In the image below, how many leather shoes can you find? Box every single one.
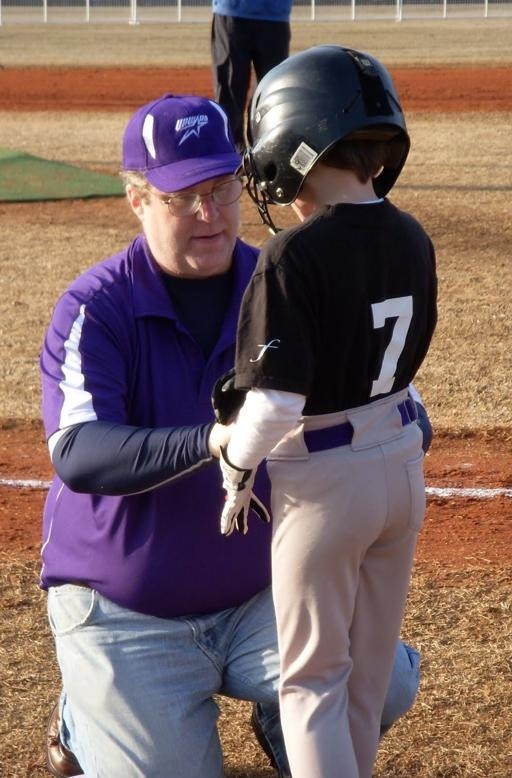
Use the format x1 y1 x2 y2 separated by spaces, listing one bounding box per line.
43 696 84 778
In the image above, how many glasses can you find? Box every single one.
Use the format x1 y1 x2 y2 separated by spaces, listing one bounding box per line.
141 177 249 220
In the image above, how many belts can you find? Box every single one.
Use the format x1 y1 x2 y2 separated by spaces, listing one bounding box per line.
304 396 418 451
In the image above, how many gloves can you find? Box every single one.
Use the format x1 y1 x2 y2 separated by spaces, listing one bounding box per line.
213 443 273 540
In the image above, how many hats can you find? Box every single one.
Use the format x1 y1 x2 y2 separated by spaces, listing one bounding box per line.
118 91 245 195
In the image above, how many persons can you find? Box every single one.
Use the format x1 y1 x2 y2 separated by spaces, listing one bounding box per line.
210 0 292 175
38 96 432 778
210 47 438 778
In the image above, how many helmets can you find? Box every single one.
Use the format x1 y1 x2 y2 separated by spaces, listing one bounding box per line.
240 44 414 208
